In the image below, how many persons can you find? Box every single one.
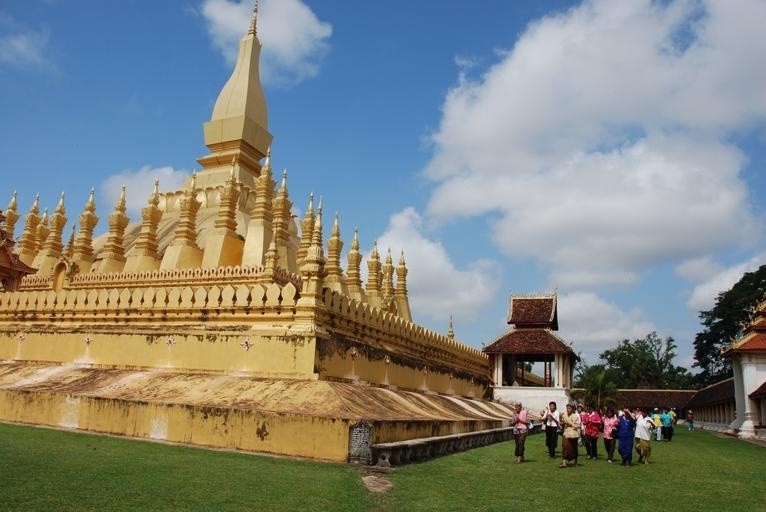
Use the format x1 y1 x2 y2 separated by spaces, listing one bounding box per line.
540 402 677 468
510 402 530 464
686 409 695 431
